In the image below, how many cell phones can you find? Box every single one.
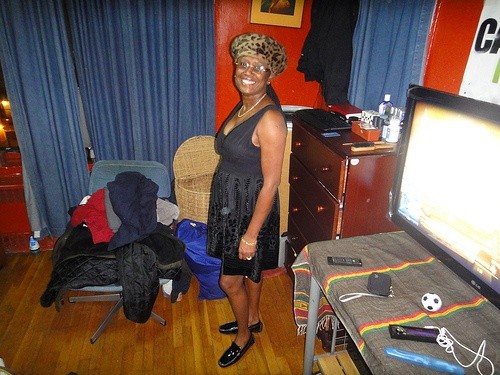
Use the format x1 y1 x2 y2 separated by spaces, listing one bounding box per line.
388 325 446 344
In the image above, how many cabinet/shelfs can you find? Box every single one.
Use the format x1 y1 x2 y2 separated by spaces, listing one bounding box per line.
0 152 94 254
291 230 500 375
284 116 398 284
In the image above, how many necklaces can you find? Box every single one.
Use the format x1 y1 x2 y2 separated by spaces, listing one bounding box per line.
238 93 267 117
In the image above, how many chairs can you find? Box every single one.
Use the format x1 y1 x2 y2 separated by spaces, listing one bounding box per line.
67 159 183 344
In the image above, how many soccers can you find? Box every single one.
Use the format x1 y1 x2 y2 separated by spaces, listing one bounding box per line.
421 292 442 311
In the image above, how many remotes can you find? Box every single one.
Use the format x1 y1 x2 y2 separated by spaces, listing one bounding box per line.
327 256 362 267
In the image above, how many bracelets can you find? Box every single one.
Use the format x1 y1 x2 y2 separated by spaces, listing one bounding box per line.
242 236 257 245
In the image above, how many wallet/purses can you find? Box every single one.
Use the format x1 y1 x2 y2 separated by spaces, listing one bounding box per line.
366 270 391 295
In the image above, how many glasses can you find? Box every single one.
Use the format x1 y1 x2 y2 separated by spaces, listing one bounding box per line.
235 59 271 74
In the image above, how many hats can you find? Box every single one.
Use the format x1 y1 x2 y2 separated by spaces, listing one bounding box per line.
230 32 288 79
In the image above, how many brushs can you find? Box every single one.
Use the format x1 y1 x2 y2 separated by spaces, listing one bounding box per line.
351 142 394 151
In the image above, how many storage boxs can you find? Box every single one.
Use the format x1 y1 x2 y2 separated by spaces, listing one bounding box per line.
352 121 379 141
361 110 380 125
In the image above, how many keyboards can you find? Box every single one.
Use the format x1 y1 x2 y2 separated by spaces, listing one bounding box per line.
294 108 353 131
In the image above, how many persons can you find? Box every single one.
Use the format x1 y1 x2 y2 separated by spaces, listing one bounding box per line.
204 32 290 368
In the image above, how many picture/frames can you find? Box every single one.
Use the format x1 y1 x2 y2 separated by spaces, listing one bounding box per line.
248 0 304 29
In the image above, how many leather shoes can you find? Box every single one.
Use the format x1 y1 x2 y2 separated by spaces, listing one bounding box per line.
218 331 256 367
218 319 263 334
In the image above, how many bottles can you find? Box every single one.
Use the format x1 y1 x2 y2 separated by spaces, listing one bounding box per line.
28 235 40 254
372 94 401 143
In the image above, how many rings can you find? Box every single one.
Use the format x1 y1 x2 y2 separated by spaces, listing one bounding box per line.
247 257 252 260
243 257 246 258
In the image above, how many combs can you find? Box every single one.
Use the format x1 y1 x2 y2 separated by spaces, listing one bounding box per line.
350 142 393 152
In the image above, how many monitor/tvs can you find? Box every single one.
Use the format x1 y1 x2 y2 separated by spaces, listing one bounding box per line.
386 83 500 311
